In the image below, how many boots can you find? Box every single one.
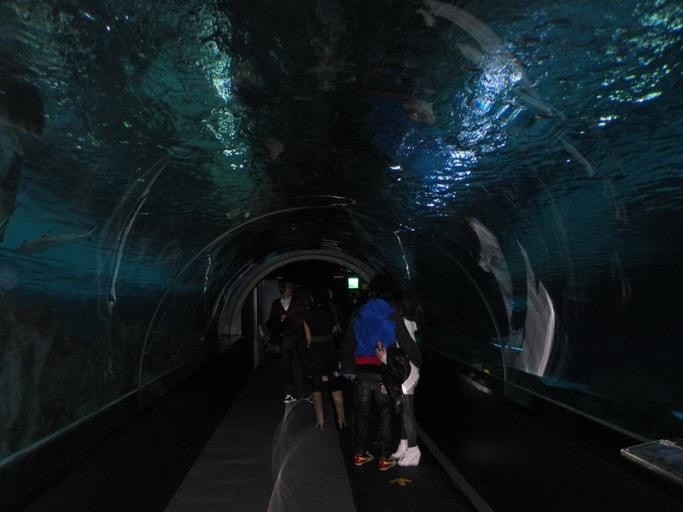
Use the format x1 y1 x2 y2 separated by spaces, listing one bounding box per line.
392 439 421 466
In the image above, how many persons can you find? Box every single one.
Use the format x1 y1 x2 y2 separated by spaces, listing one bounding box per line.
266 267 424 470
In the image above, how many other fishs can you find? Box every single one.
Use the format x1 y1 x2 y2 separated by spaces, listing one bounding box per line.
11 226 100 258
311 0 683 197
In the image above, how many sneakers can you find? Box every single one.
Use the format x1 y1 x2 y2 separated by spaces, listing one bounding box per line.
283 394 296 403
379 457 397 470
355 453 374 465
304 394 314 404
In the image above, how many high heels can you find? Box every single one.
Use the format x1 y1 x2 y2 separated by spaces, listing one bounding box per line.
338 416 347 428
315 417 324 431
